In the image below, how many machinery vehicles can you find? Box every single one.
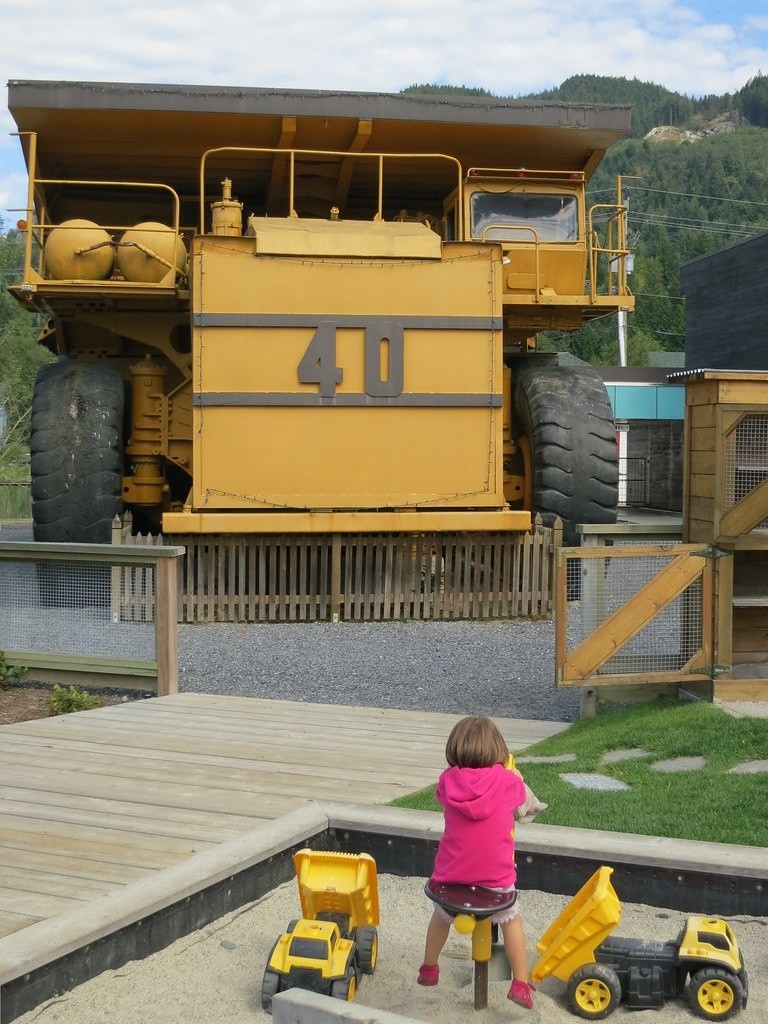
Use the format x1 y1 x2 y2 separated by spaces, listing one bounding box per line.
7 76 639 610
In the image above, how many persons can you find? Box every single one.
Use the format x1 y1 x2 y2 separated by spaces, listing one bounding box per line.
417 717 536 1009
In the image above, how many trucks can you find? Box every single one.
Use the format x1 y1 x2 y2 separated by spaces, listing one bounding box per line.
261 847 382 1005
529 866 750 1021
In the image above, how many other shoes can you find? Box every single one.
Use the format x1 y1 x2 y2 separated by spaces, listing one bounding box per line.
507 979 536 1009
417 964 440 986
